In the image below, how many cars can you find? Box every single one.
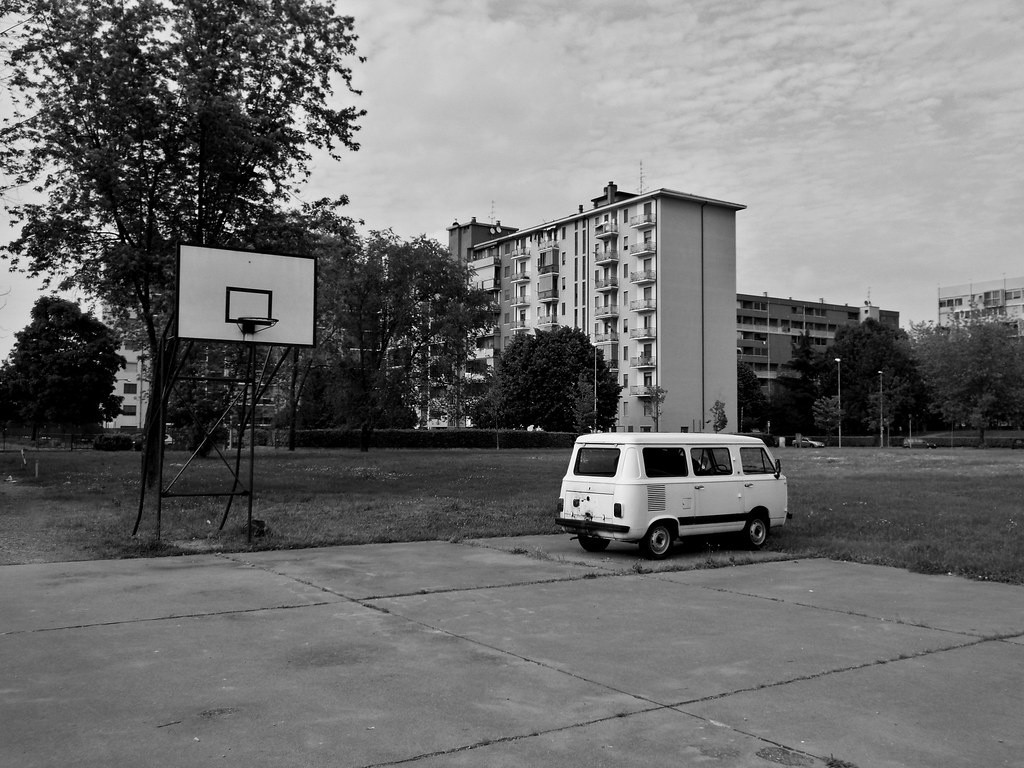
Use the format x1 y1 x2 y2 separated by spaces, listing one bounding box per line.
903 439 937 450
791 436 825 448
165 434 176 446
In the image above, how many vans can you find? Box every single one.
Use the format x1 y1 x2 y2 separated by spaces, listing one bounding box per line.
555 431 793 561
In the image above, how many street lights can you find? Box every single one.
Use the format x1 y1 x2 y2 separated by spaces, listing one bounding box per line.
878 371 885 448
835 358 842 448
591 343 598 433
